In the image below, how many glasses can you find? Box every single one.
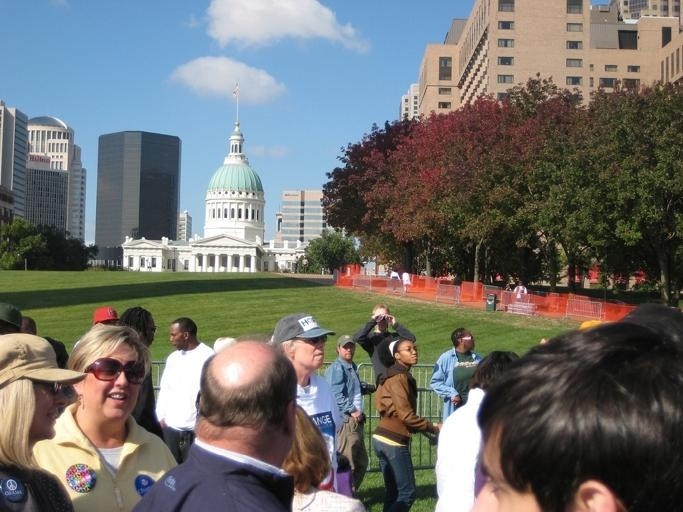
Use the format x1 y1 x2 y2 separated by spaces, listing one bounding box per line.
301 335 326 343
84 358 145 384
34 382 61 395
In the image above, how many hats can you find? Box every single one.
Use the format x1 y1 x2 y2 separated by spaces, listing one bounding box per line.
336 335 355 346
0 301 21 330
92 307 120 324
272 313 336 344
0 333 87 390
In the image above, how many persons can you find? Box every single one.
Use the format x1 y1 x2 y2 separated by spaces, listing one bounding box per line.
477 323 683 512
430 327 483 415
0 299 441 511
504 284 512 295
435 351 521 511
513 281 527 301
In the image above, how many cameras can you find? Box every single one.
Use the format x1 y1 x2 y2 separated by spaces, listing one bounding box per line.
360 381 376 395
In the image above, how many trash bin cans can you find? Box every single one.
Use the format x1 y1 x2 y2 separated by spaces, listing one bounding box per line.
486 294 496 311
